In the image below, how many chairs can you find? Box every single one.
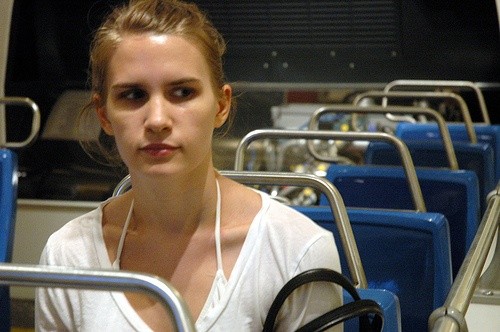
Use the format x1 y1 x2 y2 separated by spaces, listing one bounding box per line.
0 97 44 332
233 80 499 332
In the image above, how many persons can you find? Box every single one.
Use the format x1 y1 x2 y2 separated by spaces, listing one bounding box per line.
34 0 343 332
